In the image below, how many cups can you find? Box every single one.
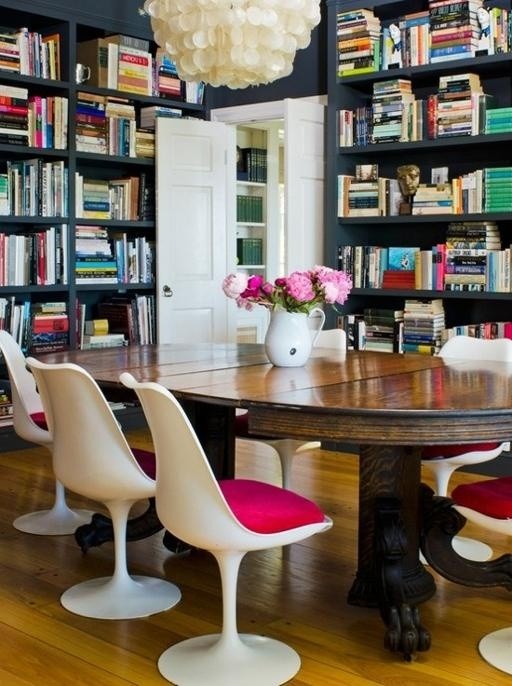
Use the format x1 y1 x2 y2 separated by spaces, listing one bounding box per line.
75 63 92 84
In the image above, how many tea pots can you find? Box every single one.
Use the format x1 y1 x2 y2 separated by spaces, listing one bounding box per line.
265 365 326 411
265 307 326 368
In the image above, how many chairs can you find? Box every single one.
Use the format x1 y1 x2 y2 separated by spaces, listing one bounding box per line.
450 475 512 677
418 335 512 567
0 328 98 536
25 356 182 620
119 372 334 686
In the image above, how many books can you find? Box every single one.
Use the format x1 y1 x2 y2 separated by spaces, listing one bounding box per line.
1 389 14 426
0 159 154 221
1 85 203 159
1 224 152 286
1 293 156 354
338 298 512 356
338 167 512 217
340 73 512 147
336 0 512 76
338 222 512 293
108 401 136 410
0 28 60 80
77 34 203 104
235 146 268 265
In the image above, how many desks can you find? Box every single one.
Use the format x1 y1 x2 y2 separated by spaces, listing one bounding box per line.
0 344 512 663
